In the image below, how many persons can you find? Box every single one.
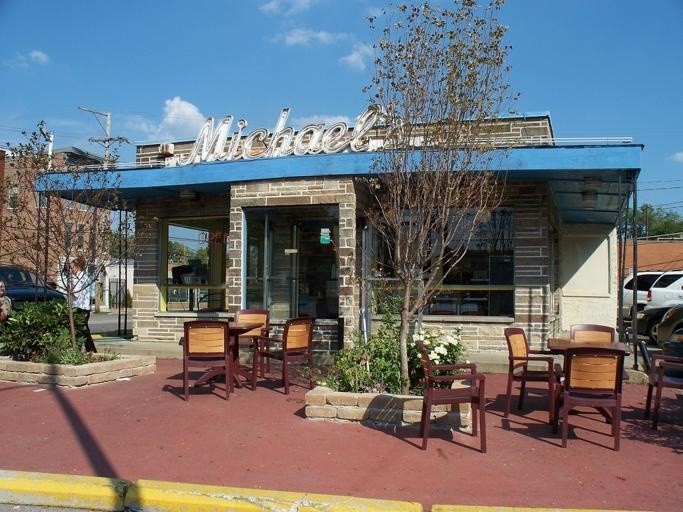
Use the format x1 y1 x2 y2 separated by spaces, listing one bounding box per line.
69 257 96 353
0 281 11 324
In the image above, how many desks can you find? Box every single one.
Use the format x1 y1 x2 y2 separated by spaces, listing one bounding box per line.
548 338 631 424
194 322 264 388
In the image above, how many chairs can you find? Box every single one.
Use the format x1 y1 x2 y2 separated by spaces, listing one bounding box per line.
504 328 558 425
563 324 629 383
429 294 459 315
236 310 273 378
552 347 625 451
179 320 235 401
645 354 683 430
251 318 316 395
415 340 486 453
459 295 489 315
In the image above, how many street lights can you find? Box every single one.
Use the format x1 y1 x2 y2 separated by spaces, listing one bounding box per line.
77 104 111 170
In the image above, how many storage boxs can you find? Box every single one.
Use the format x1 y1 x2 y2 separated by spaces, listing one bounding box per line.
471 278 490 297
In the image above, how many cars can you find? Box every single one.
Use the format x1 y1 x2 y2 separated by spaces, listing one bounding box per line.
0 264 67 309
638 307 671 342
644 270 683 309
623 271 663 321
657 304 683 356
